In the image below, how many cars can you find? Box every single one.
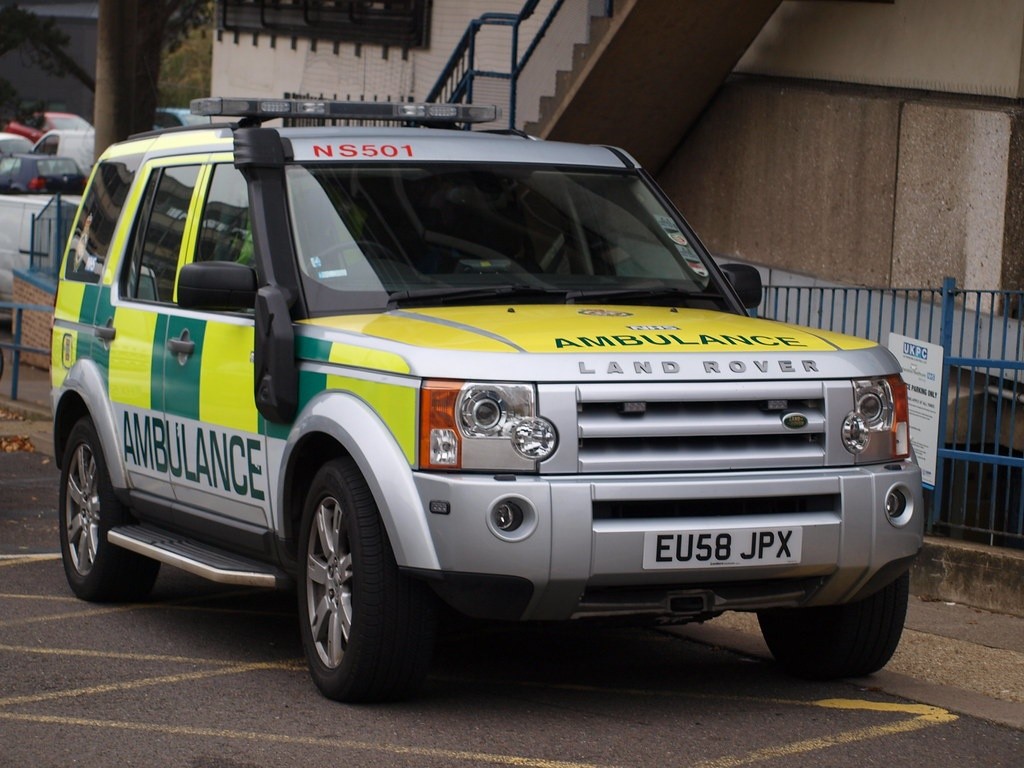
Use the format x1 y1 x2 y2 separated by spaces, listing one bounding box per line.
0 108 212 321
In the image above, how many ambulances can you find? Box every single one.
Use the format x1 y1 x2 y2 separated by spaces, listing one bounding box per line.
49 97 924 703
